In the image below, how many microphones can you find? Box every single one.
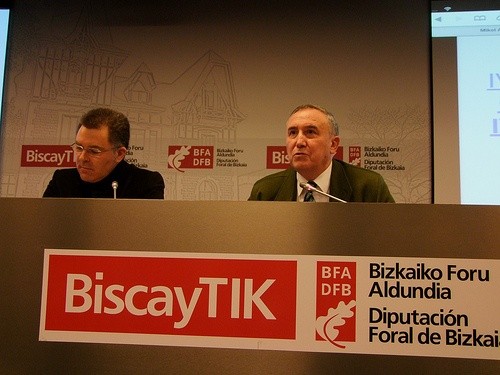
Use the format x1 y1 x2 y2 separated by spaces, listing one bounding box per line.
300 182 347 203
112 181 118 199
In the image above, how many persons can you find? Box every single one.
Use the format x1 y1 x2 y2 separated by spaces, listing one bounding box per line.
41 108 165 200
247 104 395 204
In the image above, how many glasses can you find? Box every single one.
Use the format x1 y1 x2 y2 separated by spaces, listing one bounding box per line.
69 142 121 157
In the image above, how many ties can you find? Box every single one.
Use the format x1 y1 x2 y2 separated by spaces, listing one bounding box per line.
304 181 317 202
